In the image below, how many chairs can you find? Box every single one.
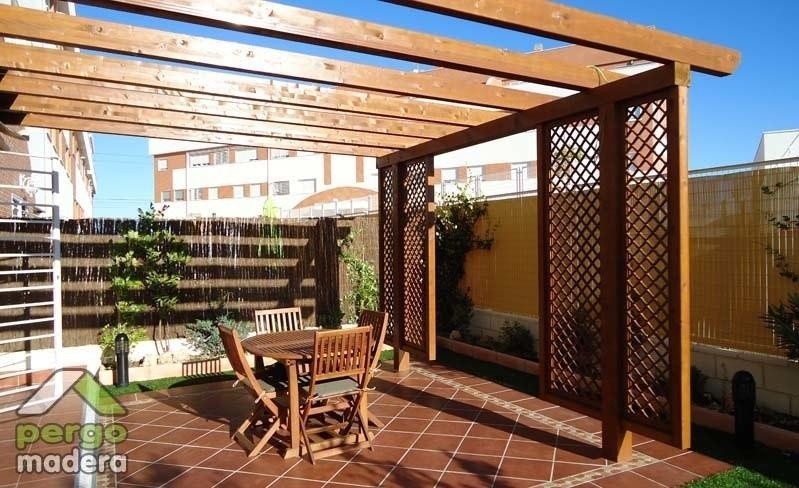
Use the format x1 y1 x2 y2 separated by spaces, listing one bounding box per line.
217 306 390 465
